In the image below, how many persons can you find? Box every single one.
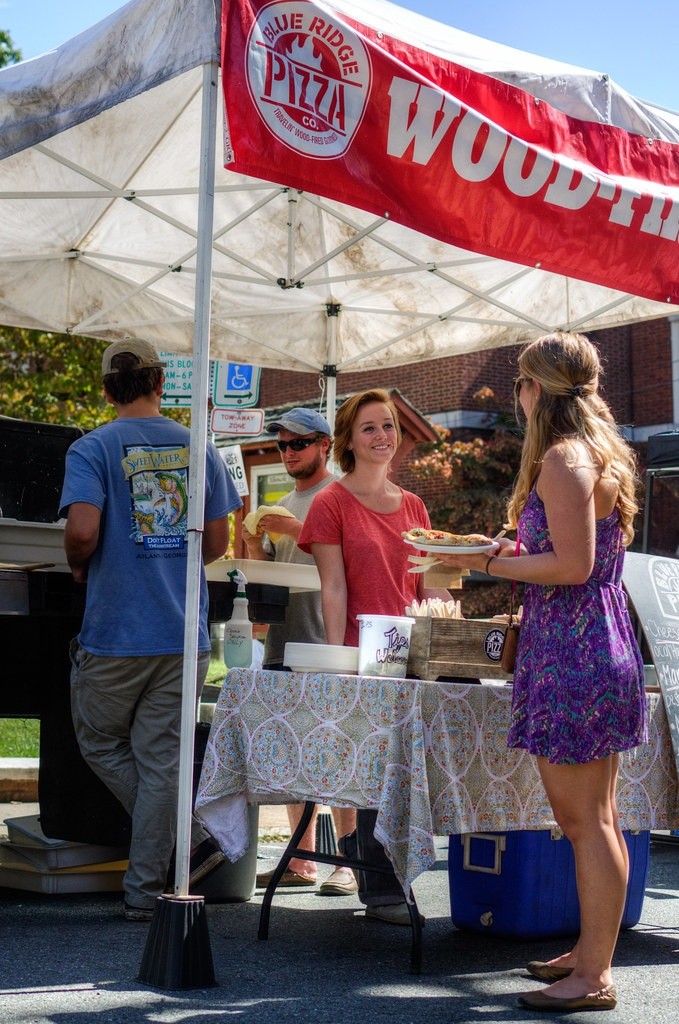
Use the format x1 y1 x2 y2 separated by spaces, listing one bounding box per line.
242 404 359 898
298 392 434 926
436 331 646 1010
59 338 243 920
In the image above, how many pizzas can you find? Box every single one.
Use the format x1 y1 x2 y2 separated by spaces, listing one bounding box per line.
405 527 494 546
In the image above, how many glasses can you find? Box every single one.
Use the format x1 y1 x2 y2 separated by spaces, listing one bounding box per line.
513 377 538 396
278 436 321 453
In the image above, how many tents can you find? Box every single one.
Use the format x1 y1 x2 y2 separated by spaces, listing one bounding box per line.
0 1 679 901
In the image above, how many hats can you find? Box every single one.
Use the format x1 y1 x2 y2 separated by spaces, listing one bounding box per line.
266 407 331 436
100 336 167 375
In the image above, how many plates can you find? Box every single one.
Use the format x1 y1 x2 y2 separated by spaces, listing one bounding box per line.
283 642 358 674
403 538 499 555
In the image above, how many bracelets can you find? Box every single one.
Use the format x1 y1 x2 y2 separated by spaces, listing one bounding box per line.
487 555 496 577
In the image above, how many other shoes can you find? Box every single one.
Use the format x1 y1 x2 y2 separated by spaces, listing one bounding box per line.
528 961 576 981
364 902 424 927
255 864 317 886
124 903 155 921
516 987 617 1014
320 869 357 895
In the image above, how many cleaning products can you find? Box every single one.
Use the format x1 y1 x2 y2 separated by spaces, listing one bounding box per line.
223 571 252 670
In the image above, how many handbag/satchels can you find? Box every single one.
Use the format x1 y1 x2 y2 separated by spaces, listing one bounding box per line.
498 625 521 675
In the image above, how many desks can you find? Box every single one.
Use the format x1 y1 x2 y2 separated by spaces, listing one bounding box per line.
194 665 679 974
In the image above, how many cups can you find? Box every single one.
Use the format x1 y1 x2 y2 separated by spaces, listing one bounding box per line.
355 614 416 679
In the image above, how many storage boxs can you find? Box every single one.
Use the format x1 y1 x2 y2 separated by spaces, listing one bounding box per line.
448 827 650 936
410 616 521 679
